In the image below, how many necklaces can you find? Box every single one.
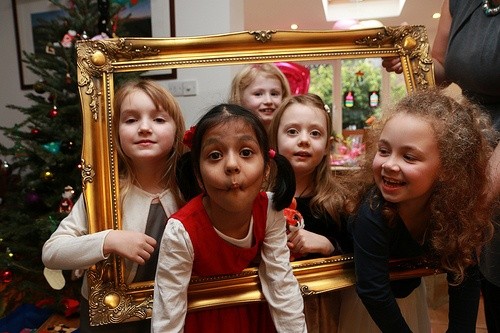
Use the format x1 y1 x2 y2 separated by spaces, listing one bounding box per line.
482 0 500 15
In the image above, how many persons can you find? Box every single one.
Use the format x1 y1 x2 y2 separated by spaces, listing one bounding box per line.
337 92 494 333
41 81 190 333
381 0 500 333
263 94 359 333
230 62 290 129
151 104 309 333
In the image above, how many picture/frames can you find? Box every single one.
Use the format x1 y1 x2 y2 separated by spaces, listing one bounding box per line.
11 0 178 91
72 24 455 326
